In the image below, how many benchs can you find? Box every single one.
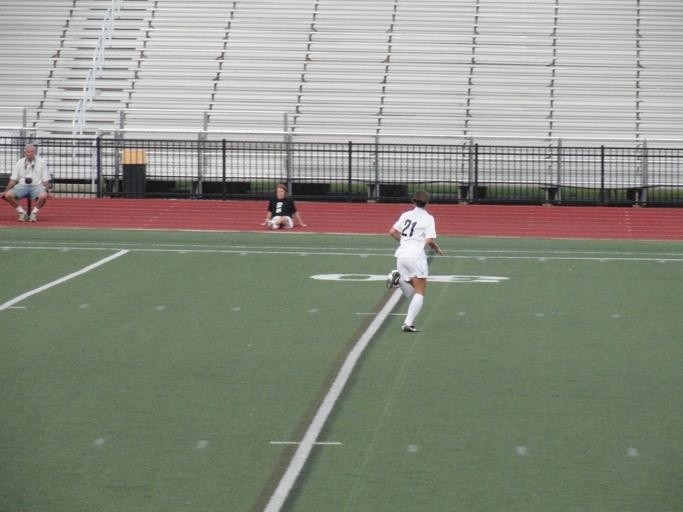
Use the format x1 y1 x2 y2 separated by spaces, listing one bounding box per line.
0 0 683 192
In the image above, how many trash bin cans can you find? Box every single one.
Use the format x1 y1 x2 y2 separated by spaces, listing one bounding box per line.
123 150 147 199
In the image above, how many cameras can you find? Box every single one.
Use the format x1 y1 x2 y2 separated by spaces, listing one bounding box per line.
25 178 32 184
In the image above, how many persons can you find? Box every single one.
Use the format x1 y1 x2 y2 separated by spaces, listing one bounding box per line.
384 190 443 332
0 144 52 223
260 184 308 230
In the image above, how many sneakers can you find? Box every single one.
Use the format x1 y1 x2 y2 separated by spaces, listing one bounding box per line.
387 270 400 288
402 324 416 331
267 220 275 230
18 213 38 222
